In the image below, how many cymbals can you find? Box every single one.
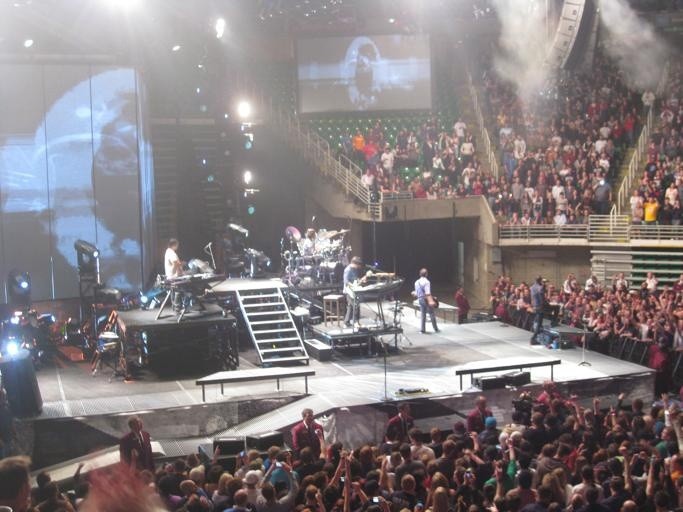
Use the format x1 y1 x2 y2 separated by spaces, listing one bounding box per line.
286 227 301 241
333 229 351 238
318 231 338 241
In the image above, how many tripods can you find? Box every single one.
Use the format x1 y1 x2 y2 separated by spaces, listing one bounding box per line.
386 295 413 348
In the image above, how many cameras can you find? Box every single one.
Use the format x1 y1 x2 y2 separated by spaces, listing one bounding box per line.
372 497 379 502
340 476 344 482
275 462 282 468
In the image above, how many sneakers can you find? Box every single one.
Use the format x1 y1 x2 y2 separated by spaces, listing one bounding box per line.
531 340 540 345
419 331 440 334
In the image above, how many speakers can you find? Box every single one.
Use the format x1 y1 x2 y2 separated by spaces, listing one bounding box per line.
472 371 531 391
0 349 44 418
546 0 603 72
212 430 284 457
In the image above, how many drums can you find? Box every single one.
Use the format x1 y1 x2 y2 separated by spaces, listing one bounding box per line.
319 262 343 280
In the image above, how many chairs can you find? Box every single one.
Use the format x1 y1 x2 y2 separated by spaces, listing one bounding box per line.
309 112 681 378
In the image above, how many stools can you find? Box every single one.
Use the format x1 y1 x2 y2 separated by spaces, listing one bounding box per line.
323 295 346 327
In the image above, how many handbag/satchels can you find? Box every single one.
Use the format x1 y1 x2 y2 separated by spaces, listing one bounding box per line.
424 296 438 309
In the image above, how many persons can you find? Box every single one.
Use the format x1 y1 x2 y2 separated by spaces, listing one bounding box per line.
456 284 470 325
342 256 364 329
164 239 184 317
489 263 681 377
0 380 681 512
415 268 442 334
334 0 683 228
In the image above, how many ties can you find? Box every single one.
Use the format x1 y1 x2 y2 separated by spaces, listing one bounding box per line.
483 414 485 424
404 418 406 435
307 427 312 444
138 434 143 449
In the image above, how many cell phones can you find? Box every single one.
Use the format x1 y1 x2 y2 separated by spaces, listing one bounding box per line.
467 471 470 479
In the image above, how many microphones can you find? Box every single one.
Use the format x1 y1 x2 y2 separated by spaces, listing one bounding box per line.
205 241 213 249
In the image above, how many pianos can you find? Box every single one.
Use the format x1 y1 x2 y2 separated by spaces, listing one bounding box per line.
352 273 406 297
161 273 227 287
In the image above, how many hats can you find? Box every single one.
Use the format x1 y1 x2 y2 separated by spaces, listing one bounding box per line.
189 465 205 481
485 417 496 428
452 421 466 434
242 469 288 484
